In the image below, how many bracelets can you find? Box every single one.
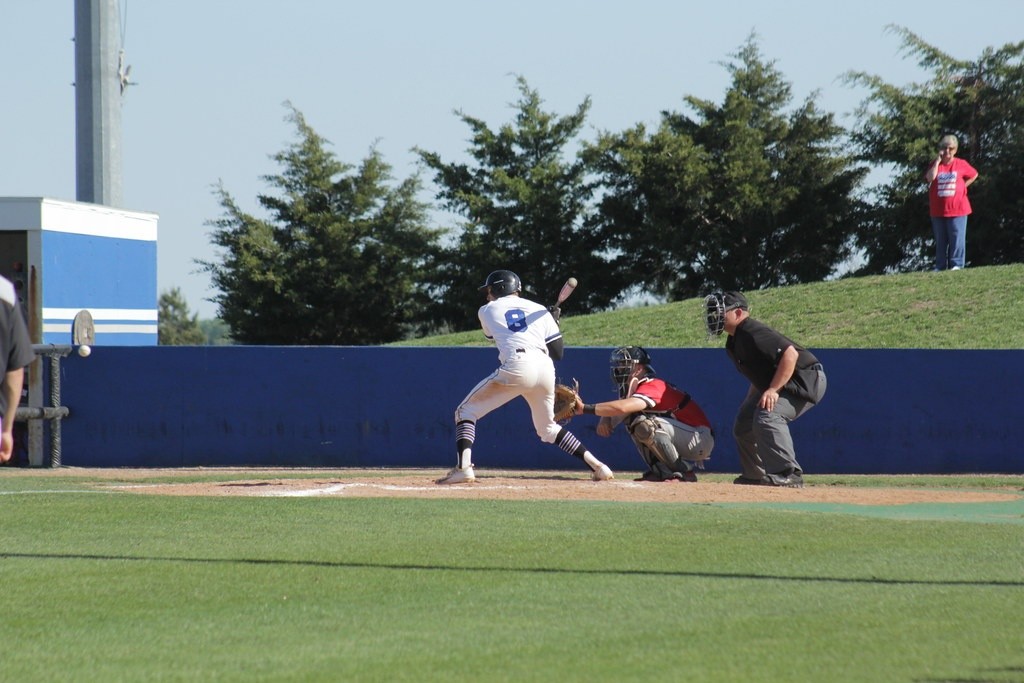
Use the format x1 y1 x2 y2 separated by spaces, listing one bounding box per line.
583 403 596 416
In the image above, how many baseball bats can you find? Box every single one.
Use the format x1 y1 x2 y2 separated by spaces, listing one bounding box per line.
554 277 578 307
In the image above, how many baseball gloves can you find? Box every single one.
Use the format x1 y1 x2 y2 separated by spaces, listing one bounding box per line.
553 376 580 422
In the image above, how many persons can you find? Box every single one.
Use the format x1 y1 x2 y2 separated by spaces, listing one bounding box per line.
701 290 827 489
924 134 978 273
553 346 715 483
0 274 36 461
434 270 614 485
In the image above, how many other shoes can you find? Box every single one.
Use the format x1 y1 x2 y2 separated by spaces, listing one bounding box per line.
931 268 940 272
951 266 960 271
666 456 697 482
634 456 672 481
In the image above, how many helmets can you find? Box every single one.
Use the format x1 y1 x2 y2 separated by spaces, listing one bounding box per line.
478 270 521 298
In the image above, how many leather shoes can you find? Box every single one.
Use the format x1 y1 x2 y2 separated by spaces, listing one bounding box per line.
516 348 547 354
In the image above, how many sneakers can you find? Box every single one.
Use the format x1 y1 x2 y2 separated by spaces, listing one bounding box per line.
760 467 803 488
734 474 770 485
592 464 614 482
435 464 475 484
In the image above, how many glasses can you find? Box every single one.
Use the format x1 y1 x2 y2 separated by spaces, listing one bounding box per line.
942 146 956 150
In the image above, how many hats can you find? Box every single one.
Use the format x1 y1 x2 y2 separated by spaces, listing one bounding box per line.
707 291 748 314
614 346 656 375
